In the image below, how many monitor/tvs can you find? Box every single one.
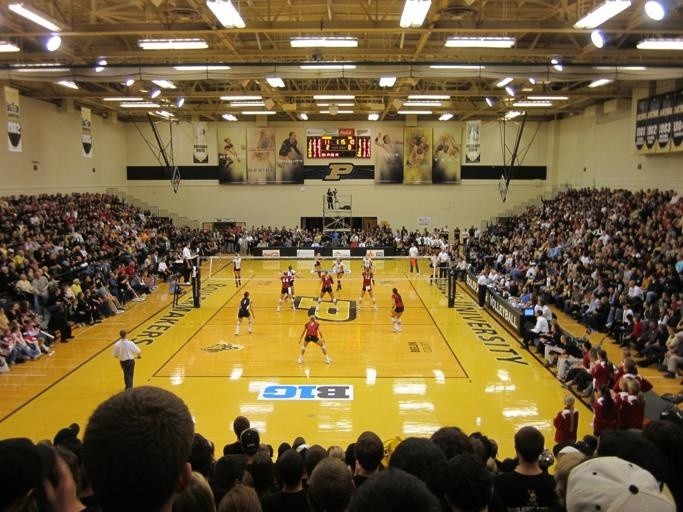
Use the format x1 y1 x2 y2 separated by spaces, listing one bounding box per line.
524 308 535 318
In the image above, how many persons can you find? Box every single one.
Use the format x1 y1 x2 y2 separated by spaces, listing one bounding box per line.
408 141 427 169
275 131 301 169
297 313 331 366
374 131 403 173
233 291 257 334
326 187 335 210
1 386 683 511
222 136 240 170
436 187 683 396
387 284 407 333
1 193 200 377
113 330 144 391
201 222 434 309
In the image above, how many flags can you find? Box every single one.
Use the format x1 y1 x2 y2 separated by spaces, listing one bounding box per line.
191 120 209 164
632 89 683 154
3 85 24 153
80 106 94 160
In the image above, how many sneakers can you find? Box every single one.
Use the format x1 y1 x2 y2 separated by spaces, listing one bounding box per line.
234 330 240 335
247 328 252 334
633 351 675 378
520 341 540 354
326 358 332 364
556 375 590 397
607 331 629 348
298 358 303 363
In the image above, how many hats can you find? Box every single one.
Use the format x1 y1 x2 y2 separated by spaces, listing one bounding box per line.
53 423 79 445
566 455 678 512
239 427 260 455
